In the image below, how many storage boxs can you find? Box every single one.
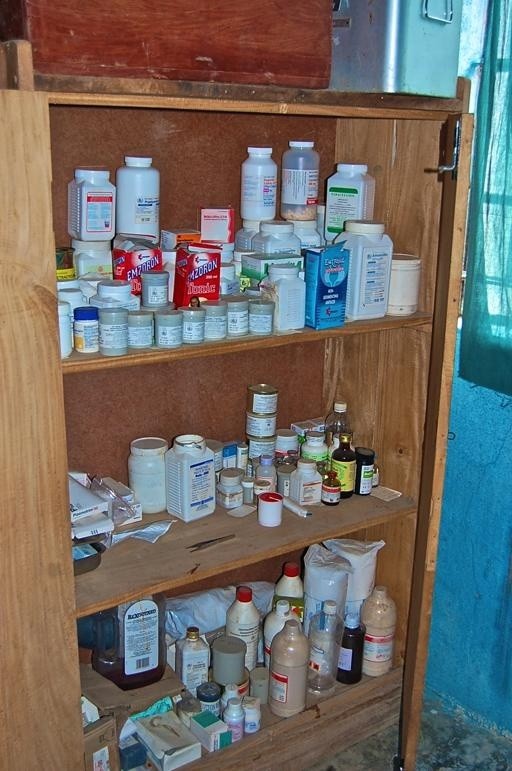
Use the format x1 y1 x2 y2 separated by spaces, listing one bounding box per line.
1 1 332 88
328 0 463 98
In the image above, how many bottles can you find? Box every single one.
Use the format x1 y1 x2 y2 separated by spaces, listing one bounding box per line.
127 383 379 527
58 142 422 357
93 562 397 741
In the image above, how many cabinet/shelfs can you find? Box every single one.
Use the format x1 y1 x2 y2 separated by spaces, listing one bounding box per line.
1 35 477 771
